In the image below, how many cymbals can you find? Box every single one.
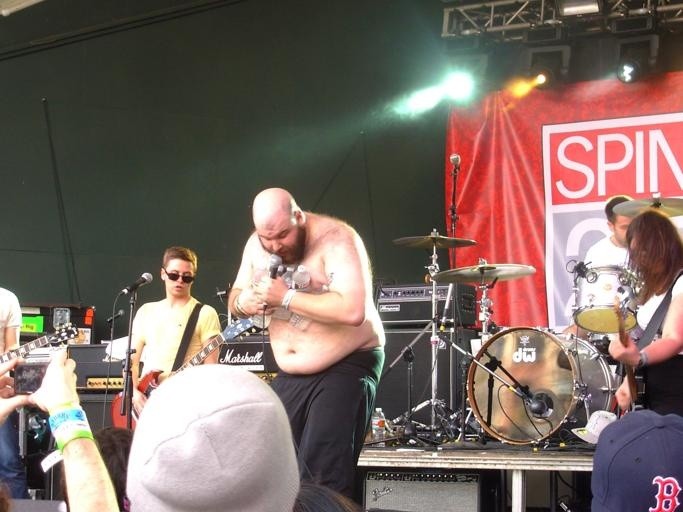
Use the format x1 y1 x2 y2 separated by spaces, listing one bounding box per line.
394 236 478 248
613 198 682 219
432 263 538 283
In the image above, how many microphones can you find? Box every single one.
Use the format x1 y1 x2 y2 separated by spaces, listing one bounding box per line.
450 153 460 169
264 254 282 311
120 272 153 295
107 309 125 322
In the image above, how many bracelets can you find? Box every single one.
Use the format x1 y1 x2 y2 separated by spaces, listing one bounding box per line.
280 289 295 309
635 351 647 368
47 402 94 454
234 297 250 318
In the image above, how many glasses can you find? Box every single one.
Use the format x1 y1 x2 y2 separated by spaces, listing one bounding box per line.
161 265 196 285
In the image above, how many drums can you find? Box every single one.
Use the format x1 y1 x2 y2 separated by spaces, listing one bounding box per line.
468 326 614 445
572 265 638 332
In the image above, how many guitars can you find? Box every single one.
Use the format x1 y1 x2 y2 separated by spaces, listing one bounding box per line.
614 298 646 411
112 318 256 432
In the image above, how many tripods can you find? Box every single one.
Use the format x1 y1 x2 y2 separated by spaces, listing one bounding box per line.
393 244 454 426
363 346 442 449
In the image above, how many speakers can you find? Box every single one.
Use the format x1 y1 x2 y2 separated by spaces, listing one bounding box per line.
46 393 114 500
375 328 475 429
362 471 502 512
67 344 143 393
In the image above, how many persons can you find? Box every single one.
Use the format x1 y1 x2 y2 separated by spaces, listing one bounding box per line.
565 194 636 307
0 286 34 501
590 409 683 512
229 186 386 502
605 207 682 422
0 351 120 511
121 247 224 414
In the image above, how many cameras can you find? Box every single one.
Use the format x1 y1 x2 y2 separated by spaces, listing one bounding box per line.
14 362 49 394
